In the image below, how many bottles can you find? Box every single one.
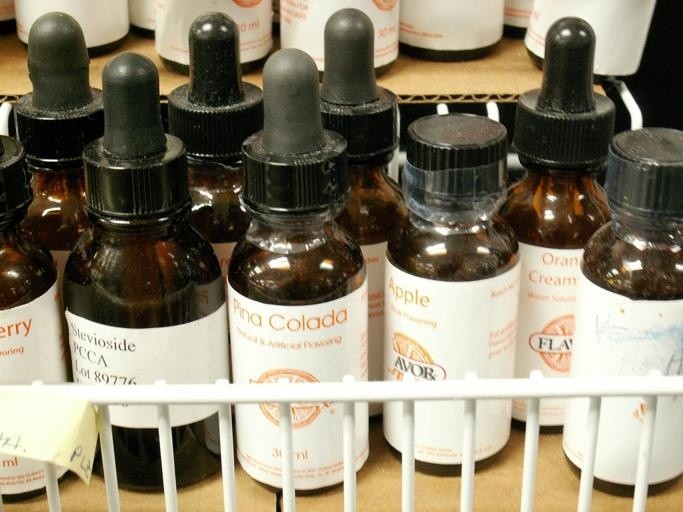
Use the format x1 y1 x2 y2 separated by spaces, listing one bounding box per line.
61 52 237 494
224 49 371 499
166 11 264 288
306 7 404 420
555 124 682 494
376 114 521 479
501 13 620 431
6 7 108 288
0 125 77 496
